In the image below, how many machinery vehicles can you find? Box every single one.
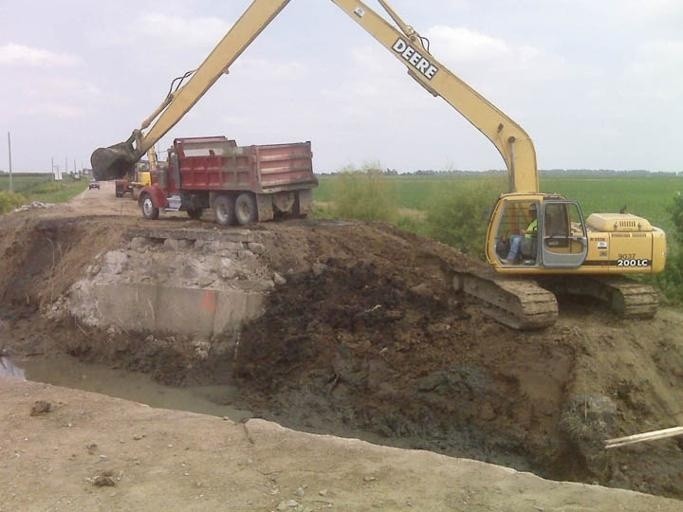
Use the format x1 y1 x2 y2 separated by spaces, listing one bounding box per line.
91 0 666 332
132 135 159 202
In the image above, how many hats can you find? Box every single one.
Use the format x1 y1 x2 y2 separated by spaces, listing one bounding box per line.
528 203 535 211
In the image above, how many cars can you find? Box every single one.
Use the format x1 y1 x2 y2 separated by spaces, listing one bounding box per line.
86 177 101 192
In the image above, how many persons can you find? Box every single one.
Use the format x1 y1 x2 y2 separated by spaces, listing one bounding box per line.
499 204 537 265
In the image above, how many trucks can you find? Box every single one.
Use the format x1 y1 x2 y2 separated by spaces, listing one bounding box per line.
112 152 146 201
138 134 320 226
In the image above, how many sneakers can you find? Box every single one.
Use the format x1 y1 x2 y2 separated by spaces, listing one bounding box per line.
499 259 512 265
